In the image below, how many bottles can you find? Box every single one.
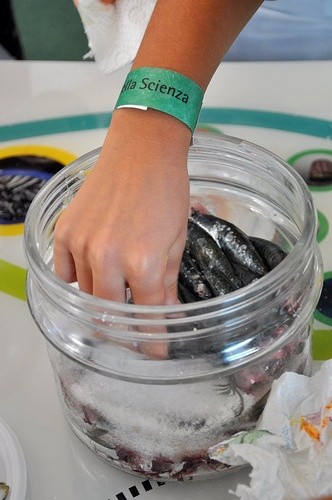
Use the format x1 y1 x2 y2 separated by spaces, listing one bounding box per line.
19 126 326 488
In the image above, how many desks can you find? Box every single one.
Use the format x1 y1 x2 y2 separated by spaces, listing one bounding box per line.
0 57 331 500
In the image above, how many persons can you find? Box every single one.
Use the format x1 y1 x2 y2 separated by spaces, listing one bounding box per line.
1 0 265 360
